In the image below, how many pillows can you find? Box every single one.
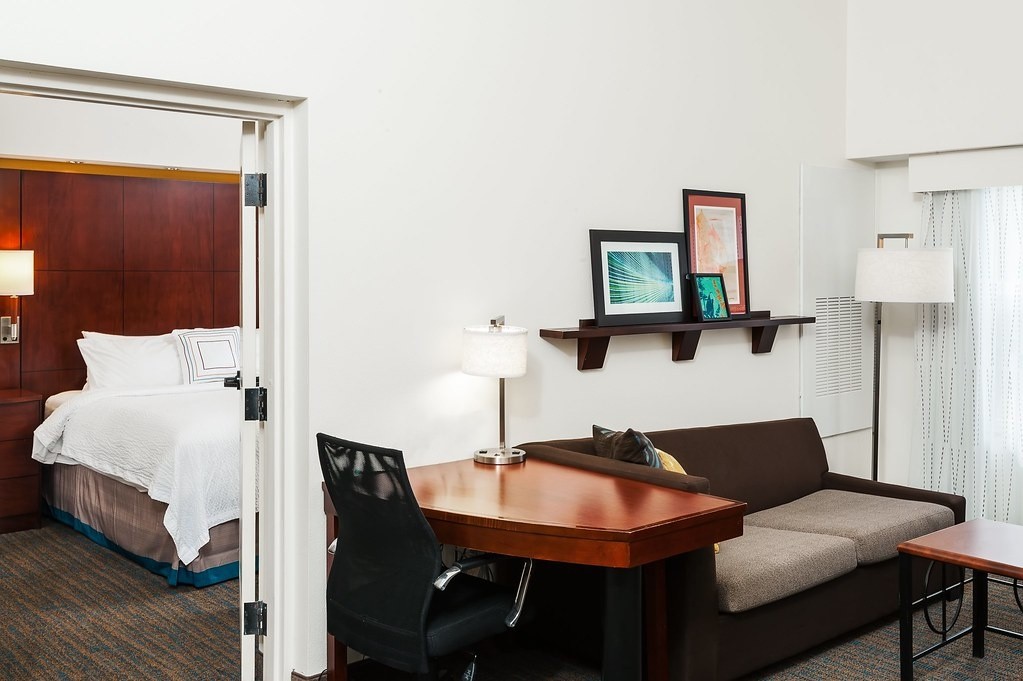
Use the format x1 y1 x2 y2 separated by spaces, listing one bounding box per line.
76 325 259 394
592 424 664 468
654 446 720 553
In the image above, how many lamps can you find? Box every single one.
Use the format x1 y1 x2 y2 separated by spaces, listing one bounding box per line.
855 232 958 480
462 314 528 464
0 249 35 343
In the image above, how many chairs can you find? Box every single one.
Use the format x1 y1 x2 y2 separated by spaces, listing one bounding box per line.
314 431 534 681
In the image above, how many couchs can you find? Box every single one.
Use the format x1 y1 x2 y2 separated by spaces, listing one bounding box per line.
510 415 967 681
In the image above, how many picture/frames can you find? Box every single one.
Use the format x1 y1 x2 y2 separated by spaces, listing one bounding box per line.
693 271 732 321
589 228 698 326
681 188 751 319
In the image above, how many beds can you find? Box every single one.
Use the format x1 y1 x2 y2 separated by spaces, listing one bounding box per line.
29 389 261 589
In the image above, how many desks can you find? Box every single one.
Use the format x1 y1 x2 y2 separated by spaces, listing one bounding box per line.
321 458 749 681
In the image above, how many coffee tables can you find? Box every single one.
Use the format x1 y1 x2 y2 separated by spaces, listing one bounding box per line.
895 517 1023 680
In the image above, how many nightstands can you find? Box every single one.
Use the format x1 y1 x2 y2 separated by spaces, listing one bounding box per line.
0 387 44 533
